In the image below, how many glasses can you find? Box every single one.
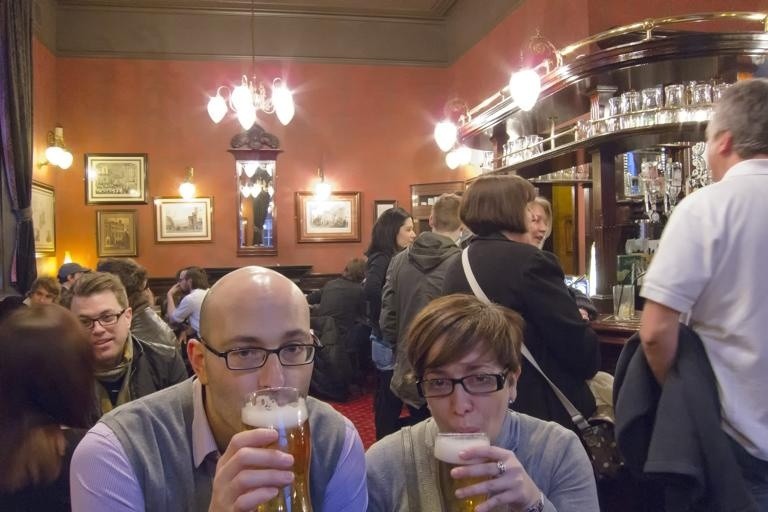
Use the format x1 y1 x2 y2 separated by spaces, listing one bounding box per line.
197 330 325 372
77 307 125 331
414 365 512 400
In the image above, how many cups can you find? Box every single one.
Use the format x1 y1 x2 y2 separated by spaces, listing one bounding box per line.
612 285 634 320
434 433 492 512
242 386 313 510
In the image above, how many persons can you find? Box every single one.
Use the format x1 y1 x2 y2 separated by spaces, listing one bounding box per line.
638 78 767 510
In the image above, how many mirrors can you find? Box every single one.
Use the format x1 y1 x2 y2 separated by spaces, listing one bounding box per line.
225 124 286 259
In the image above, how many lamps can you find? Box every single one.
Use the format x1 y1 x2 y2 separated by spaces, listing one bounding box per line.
312 166 333 202
202 1 299 133
237 160 276 197
177 166 201 202
506 51 549 114
434 98 469 155
36 121 75 174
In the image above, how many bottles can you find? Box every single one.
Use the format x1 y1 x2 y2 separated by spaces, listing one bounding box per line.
502 136 590 180
576 81 730 140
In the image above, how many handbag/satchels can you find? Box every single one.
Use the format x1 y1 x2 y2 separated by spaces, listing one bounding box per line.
577 404 627 492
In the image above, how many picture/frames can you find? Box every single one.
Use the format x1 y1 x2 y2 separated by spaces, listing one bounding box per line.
81 151 150 207
95 208 139 260
29 178 60 259
151 195 215 245
373 198 397 228
294 190 361 244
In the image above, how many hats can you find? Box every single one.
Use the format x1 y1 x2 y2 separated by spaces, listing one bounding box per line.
57 262 91 279
